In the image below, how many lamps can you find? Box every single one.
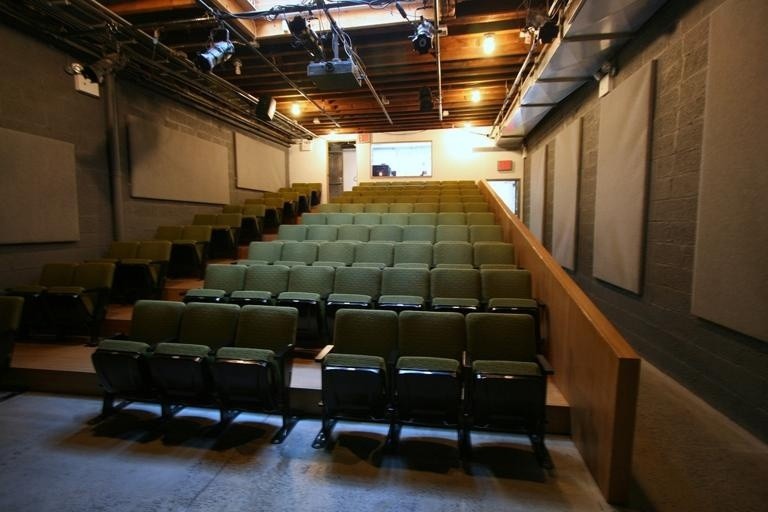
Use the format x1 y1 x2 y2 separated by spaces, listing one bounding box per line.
594 62 616 100
72 61 101 98
256 95 277 121
198 19 236 72
420 86 434 113
395 2 437 54
84 47 129 84
523 3 559 44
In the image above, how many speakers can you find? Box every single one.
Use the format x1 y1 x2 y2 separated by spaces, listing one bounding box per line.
254 95 278 122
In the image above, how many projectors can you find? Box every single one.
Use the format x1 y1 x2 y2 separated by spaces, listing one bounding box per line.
308 59 362 94
